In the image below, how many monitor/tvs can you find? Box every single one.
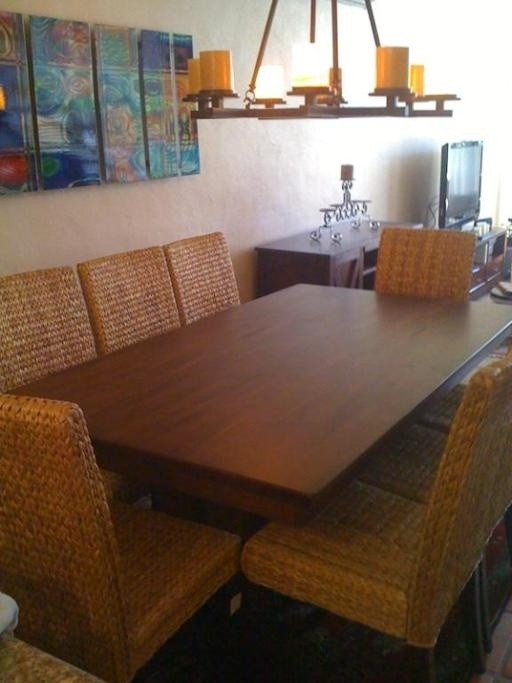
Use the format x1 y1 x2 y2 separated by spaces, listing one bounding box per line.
441 142 483 232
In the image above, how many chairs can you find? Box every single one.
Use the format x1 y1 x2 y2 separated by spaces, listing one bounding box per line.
1 263 151 503
77 244 185 352
162 231 241 325
422 338 511 431
0 392 241 683
243 350 512 683
377 225 477 299
342 421 512 654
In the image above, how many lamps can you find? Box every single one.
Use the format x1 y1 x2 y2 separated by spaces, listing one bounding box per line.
181 0 462 120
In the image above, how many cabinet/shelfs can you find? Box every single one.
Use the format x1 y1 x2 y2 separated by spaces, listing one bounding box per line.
252 218 509 302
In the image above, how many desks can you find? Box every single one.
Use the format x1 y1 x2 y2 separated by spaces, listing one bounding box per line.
11 280 512 531
